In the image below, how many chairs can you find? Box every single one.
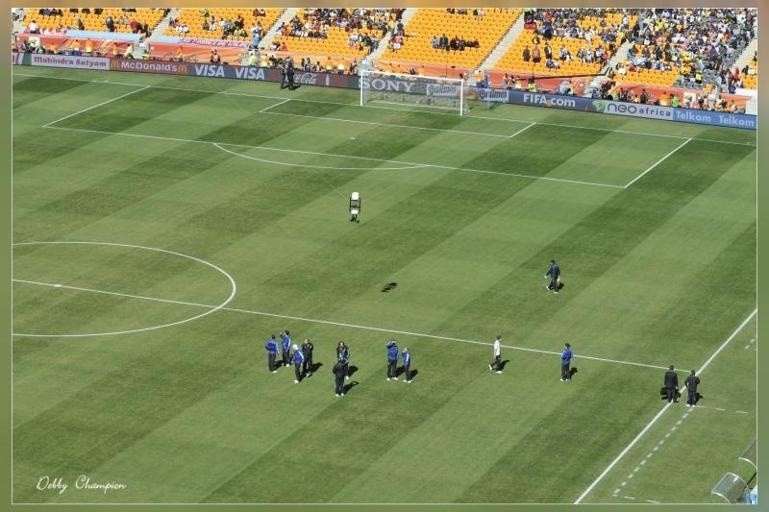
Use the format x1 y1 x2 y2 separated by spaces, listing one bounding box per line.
12 9 757 116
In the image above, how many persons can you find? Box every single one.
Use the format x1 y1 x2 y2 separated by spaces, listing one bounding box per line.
285 330 292 362
279 329 291 367
336 341 350 380
545 259 561 294
291 344 304 384
301 338 313 376
265 334 280 373
664 365 679 402
386 341 400 381
488 336 503 374
402 347 413 383
332 360 345 396
685 370 700 405
560 343 573 382
